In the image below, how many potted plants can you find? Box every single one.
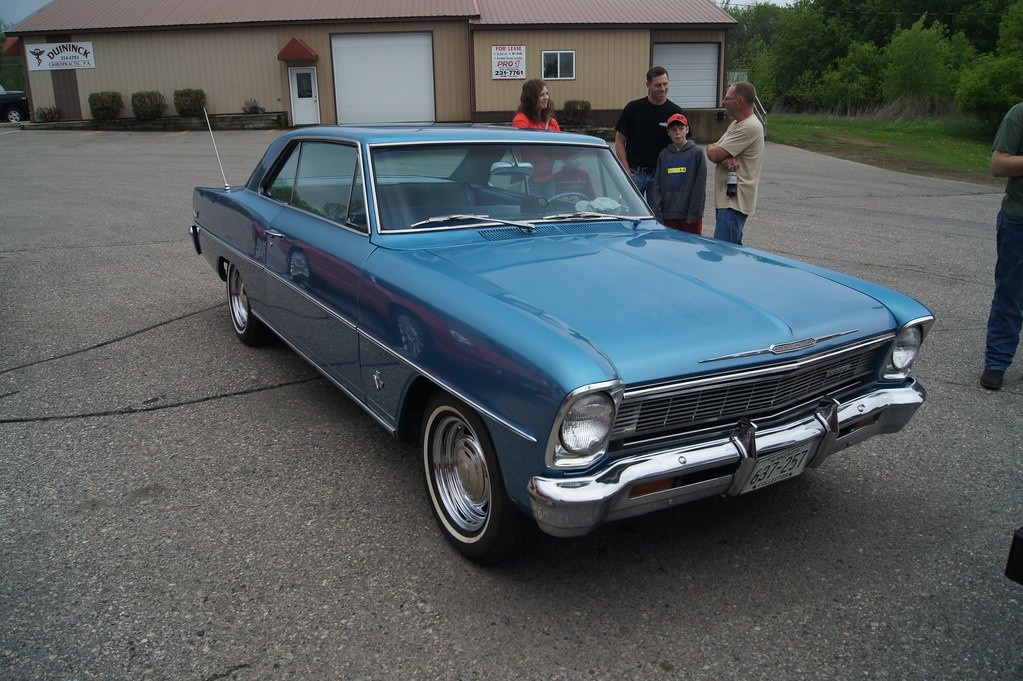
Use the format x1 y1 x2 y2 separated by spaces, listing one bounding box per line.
240 98 265 114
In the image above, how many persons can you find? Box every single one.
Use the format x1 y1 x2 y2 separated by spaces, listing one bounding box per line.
654 113 707 235
615 66 692 224
706 81 764 245
512 78 597 203
979 101 1023 390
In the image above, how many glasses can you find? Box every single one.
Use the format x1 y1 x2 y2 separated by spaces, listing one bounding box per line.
722 95 739 102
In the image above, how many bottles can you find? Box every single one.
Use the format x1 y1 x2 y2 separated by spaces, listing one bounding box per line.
726 164 737 197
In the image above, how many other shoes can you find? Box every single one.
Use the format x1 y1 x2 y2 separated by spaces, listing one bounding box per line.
980 369 1004 389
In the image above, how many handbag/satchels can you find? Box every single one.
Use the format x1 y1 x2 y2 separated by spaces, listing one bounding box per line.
504 175 530 195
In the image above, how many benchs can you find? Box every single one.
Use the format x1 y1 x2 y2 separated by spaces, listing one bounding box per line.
273 181 488 233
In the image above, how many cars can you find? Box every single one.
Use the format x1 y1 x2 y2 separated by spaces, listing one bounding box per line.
186 124 937 570
0 84 30 123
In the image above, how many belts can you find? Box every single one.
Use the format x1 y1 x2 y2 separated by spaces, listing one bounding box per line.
629 164 656 175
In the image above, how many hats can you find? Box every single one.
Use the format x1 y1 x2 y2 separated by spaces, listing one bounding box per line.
667 113 688 127
575 197 622 216
